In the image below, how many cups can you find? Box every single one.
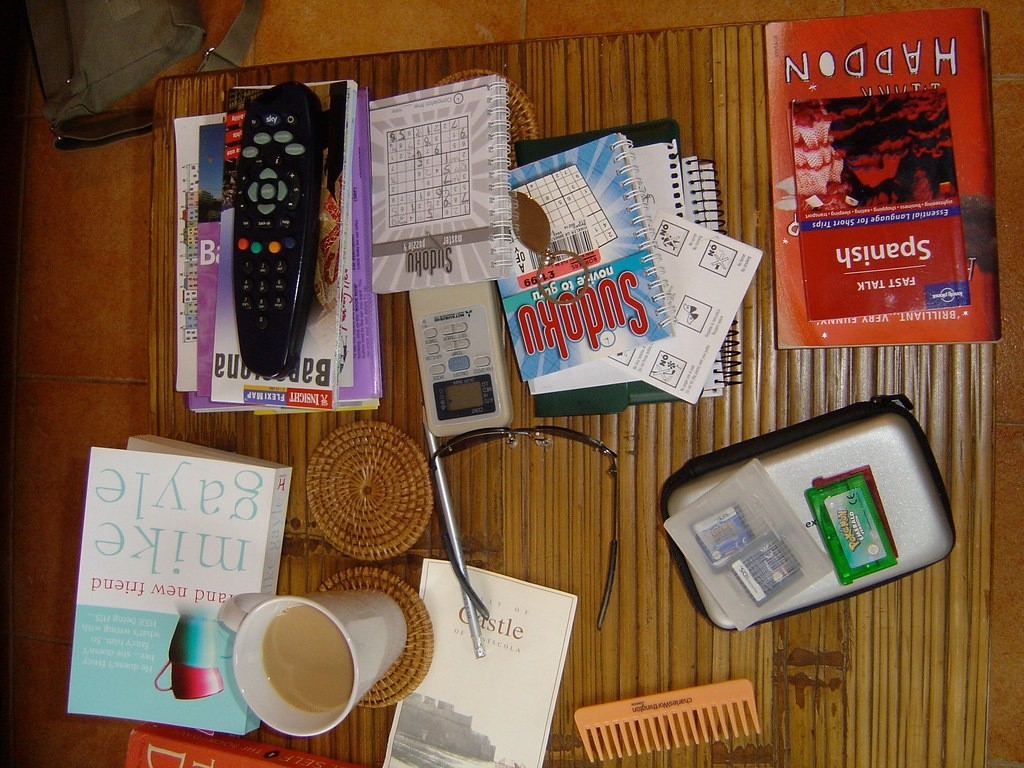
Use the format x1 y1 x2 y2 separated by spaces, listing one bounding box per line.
154 660 223 700
169 616 233 668
221 590 407 737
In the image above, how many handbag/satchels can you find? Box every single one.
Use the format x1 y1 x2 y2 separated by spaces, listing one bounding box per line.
25 0 265 154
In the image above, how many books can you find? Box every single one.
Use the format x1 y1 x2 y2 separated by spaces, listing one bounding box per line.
172 79 383 414
764 9 1002 346
65 435 293 732
531 141 743 407
789 87 970 321
126 723 364 768
498 133 676 382
370 75 515 296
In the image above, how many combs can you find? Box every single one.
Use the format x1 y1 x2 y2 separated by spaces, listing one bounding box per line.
575 680 760 761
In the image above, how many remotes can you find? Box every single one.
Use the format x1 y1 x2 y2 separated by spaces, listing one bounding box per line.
235 79 325 372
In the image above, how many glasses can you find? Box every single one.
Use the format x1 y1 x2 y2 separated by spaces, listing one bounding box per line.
427 423 622 631
